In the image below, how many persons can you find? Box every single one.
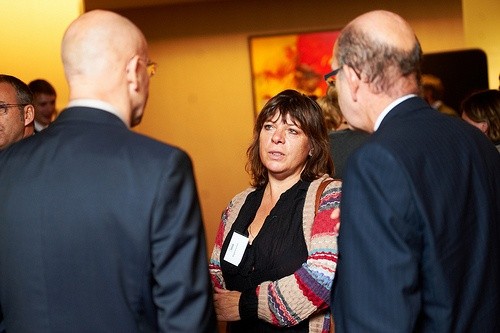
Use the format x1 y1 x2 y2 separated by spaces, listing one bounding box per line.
28 78 57 135
0 74 36 148
419 73 500 153
308 79 379 181
331 10 500 333
0 9 219 333
208 89 342 333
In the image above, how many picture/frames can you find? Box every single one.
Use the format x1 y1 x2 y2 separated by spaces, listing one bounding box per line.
246 27 345 120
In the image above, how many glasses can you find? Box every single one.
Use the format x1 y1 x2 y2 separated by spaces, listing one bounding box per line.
139 56 157 78
320 64 343 83
0 102 28 114
